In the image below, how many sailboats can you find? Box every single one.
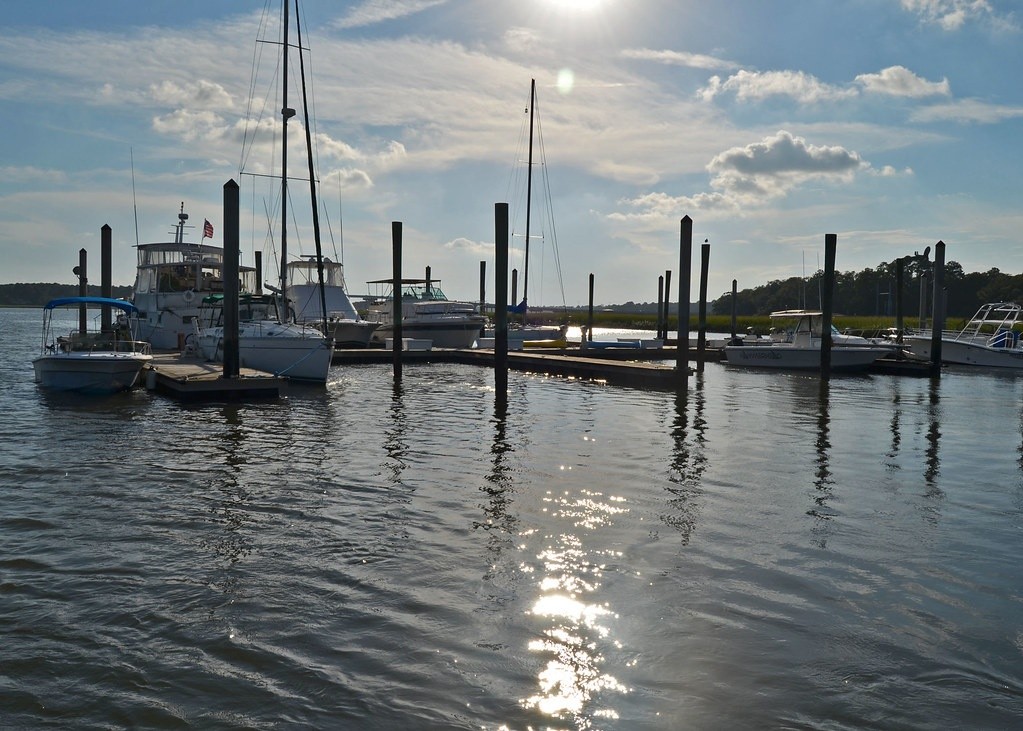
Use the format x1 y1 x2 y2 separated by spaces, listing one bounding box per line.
201 0 385 348
478 78 569 347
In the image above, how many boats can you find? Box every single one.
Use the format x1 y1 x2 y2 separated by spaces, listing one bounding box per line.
32 297 153 405
883 301 1023 368
118 146 333 382
724 251 890 368
357 278 488 348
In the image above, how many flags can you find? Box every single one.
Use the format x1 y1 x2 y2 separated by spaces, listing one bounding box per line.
204 220 214 238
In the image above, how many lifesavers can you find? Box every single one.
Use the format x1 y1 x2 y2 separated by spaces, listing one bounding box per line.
183 290 196 303
